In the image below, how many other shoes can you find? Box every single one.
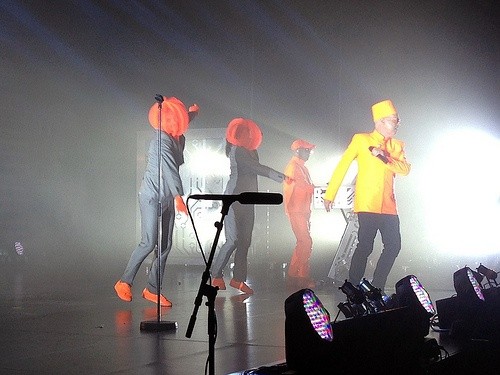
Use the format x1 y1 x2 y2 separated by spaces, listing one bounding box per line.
212 278 226 290
142 288 172 306
115 280 132 302
288 278 315 288
230 279 253 294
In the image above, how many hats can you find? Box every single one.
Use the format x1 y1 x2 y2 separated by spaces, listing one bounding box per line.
292 140 316 151
372 100 397 122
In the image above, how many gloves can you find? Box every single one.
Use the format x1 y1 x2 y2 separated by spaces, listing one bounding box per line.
190 104 199 114
176 196 188 216
285 177 295 185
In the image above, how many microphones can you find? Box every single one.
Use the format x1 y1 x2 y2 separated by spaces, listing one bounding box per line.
369 146 389 163
189 192 283 205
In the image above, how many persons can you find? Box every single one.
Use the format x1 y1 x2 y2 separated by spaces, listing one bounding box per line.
113 93 199 307
209 118 296 295
323 98 412 299
282 137 322 289
